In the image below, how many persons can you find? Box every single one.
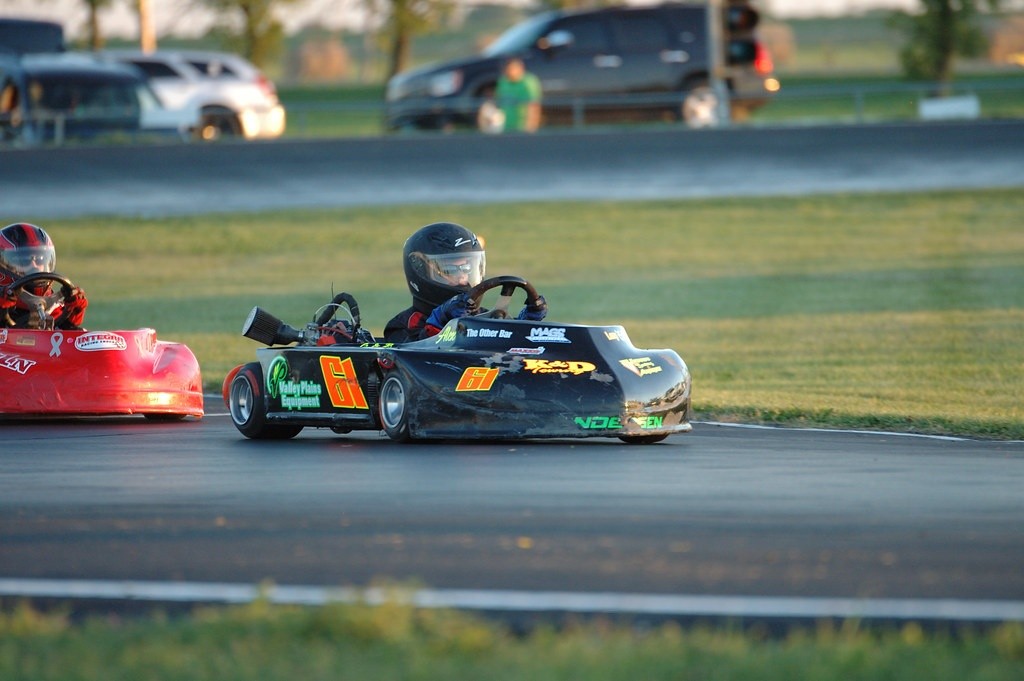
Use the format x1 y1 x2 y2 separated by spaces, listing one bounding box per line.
0 222 89 330
493 58 543 130
381 220 549 343
679 68 720 128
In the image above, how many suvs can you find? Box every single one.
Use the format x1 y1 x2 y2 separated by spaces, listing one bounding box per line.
384 2 769 132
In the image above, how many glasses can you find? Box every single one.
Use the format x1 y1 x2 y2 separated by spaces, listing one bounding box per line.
434 263 474 276
6 252 51 267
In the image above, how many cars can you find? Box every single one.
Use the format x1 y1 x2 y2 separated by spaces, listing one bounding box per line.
0 36 284 155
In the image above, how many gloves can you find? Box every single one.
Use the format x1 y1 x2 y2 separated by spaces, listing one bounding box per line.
516 296 547 321
425 293 488 331
0 285 17 316
58 286 88 330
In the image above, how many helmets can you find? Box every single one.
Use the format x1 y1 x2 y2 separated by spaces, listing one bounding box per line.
0 222 56 296
402 222 486 307
